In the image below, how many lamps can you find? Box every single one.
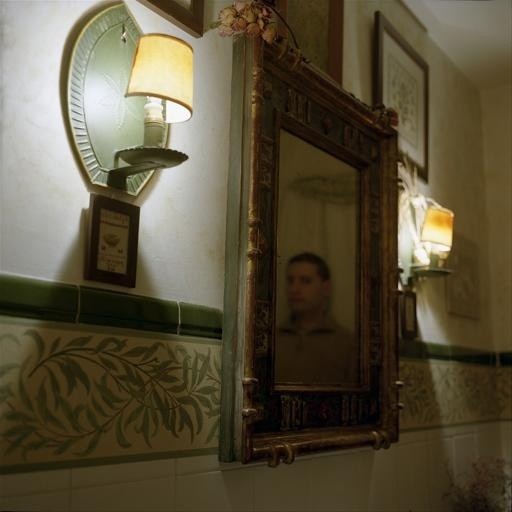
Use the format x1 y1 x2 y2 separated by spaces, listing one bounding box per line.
105 33 200 193
405 203 459 283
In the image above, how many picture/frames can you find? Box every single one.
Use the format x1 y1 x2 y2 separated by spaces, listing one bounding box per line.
217 26 403 469
135 0 214 40
370 11 431 186
82 194 148 290
398 288 423 335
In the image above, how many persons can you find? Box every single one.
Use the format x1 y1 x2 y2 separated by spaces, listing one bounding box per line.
274 251 362 383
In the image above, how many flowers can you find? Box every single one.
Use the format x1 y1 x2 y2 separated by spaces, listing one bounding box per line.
219 4 301 55
